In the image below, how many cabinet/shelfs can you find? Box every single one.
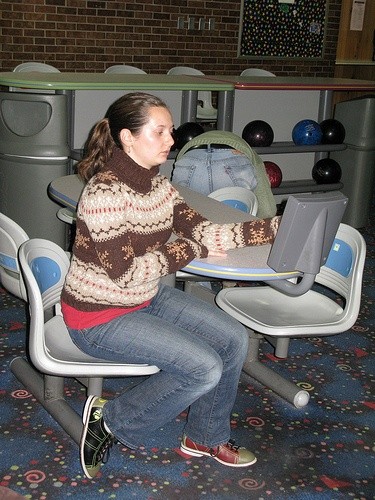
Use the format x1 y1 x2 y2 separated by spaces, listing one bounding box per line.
52 78 346 191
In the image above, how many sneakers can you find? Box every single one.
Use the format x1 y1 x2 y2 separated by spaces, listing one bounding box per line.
180 431 257 467
80 394 118 480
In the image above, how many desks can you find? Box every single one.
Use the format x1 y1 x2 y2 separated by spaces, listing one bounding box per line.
44 167 349 410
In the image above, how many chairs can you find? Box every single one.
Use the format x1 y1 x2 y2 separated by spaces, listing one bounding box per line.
240 68 276 77
0 211 73 300
216 223 366 409
168 67 218 122
170 186 259 284
10 62 66 97
10 237 161 452
105 65 148 75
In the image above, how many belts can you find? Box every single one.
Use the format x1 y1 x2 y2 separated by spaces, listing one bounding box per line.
185 143 235 152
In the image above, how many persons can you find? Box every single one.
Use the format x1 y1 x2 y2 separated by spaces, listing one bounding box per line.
172 130 277 288
60 92 285 479
